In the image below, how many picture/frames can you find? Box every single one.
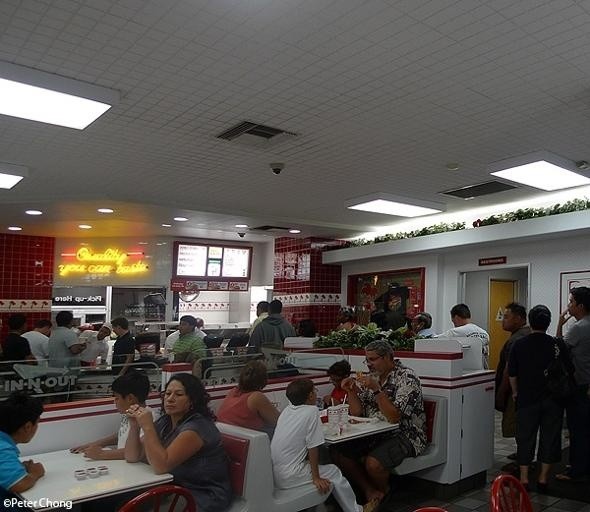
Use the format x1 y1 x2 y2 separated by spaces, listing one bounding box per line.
559 270 590 340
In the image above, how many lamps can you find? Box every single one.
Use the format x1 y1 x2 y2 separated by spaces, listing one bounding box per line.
0 60 119 132
0 163 33 191
485 150 590 192
343 193 447 218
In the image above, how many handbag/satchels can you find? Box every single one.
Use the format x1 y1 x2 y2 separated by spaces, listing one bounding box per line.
544 337 578 406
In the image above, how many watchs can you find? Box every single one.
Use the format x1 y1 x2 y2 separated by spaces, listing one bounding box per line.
371 387 384 398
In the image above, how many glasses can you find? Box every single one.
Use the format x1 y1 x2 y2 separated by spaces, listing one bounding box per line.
363 353 385 366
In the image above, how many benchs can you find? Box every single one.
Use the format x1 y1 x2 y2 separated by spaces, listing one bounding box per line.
214 422 333 510
391 395 449 490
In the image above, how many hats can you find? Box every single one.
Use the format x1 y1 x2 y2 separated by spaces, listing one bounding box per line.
102 322 112 331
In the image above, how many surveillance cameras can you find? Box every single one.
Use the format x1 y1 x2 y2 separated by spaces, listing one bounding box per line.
237 232 245 237
271 164 282 174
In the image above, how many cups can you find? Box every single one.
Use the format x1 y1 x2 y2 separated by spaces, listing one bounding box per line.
338 404 350 434
74 464 112 480
327 407 341 436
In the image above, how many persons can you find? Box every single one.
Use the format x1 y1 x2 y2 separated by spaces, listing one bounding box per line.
371 283 411 331
433 303 490 369
0 314 38 395
323 360 351 408
248 301 269 336
0 389 45 512
494 303 530 471
48 311 87 402
110 317 136 378
78 323 112 367
171 315 207 362
20 319 53 402
407 313 435 337
509 306 575 487
336 306 357 330
340 340 427 501
271 379 379 512
163 318 207 362
556 288 590 481
217 361 280 430
124 373 233 512
247 300 297 366
299 319 315 338
70 373 163 460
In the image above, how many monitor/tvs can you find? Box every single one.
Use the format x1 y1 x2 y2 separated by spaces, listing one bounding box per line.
202 335 225 348
135 332 160 360
227 333 249 350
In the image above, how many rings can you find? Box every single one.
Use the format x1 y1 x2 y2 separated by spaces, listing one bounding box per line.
138 412 141 414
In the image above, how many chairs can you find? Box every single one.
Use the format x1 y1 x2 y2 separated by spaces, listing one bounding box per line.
412 506 448 512
118 485 199 512
489 475 536 512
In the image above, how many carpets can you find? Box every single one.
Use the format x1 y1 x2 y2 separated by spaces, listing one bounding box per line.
490 446 590 503
